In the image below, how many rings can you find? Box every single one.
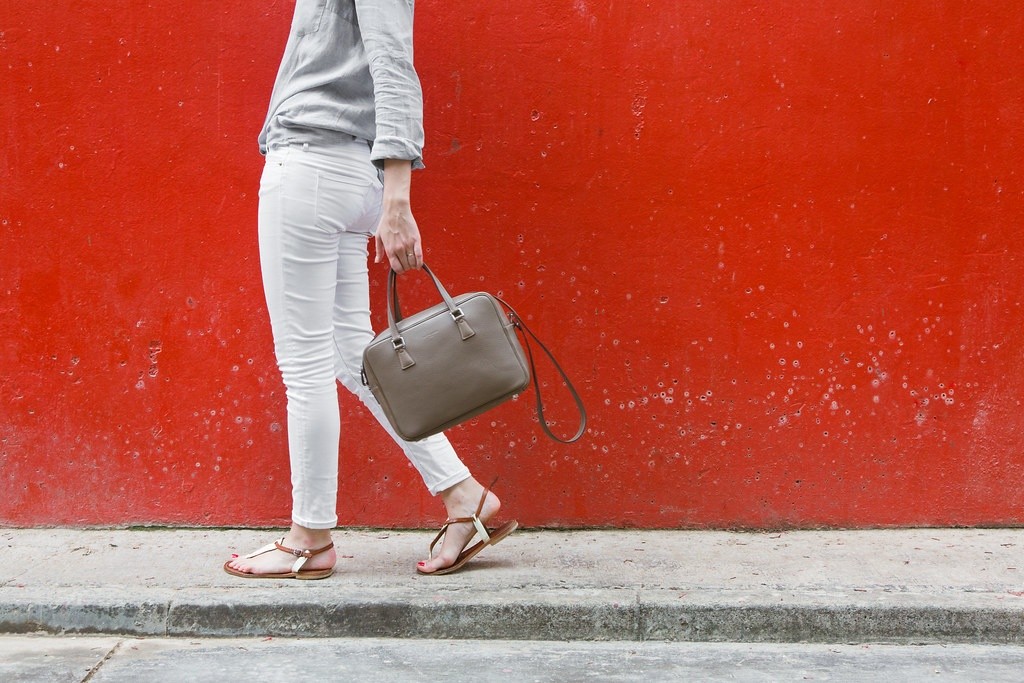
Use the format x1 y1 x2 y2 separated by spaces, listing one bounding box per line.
407 254 413 256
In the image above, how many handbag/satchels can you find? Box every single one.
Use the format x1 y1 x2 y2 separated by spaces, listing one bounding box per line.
359 258 587 443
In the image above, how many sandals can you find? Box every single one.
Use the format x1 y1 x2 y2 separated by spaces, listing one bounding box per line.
224 536 337 580
418 474 518 575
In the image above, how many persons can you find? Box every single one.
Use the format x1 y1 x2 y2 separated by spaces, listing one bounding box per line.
224 0 518 580
